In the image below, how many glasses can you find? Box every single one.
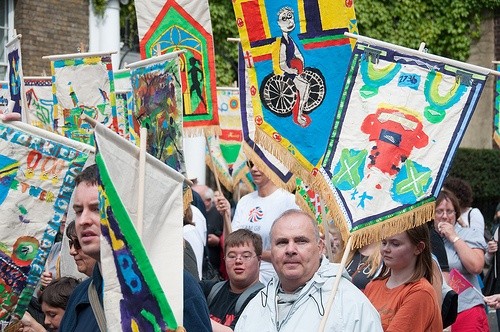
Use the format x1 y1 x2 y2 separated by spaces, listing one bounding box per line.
69 238 81 250
246 160 254 168
226 254 257 261
435 210 456 216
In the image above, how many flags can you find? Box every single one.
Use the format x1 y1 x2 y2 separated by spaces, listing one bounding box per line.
446 268 471 294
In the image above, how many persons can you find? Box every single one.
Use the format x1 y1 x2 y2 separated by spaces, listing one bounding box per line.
19 160 500 332
0 108 20 122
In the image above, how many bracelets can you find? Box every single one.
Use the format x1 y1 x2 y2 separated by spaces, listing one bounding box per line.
451 237 461 244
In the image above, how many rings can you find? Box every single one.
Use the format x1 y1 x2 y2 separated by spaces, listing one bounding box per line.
220 205 222 208
